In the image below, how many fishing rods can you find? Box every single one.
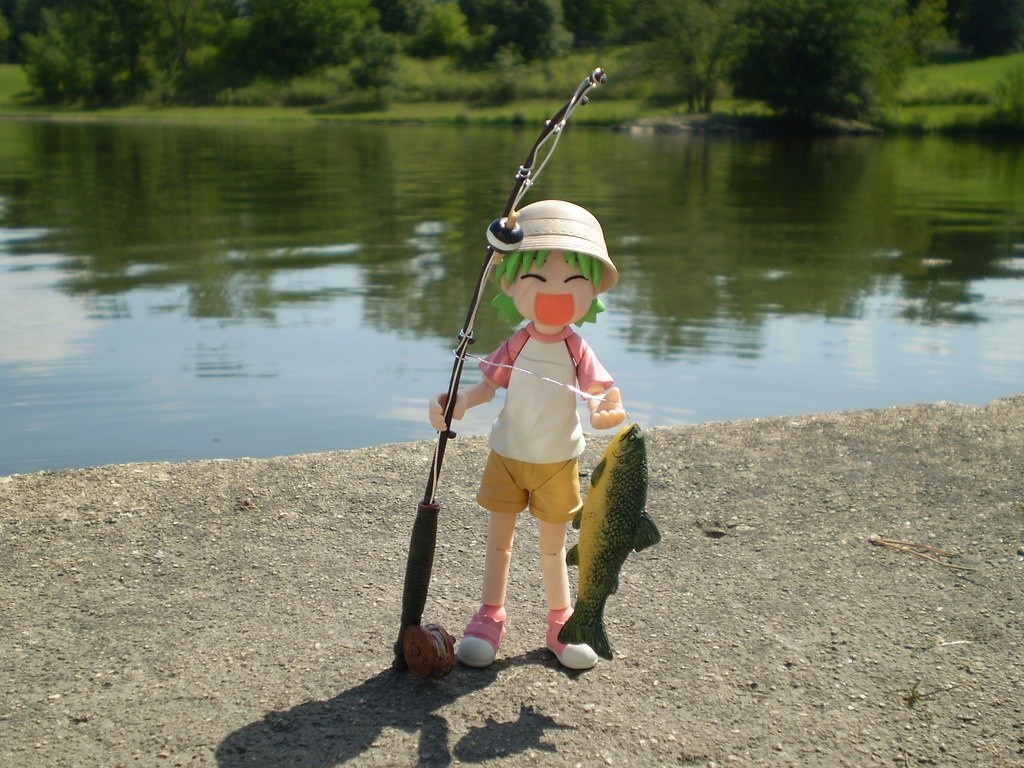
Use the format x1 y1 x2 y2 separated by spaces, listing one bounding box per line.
388 67 610 672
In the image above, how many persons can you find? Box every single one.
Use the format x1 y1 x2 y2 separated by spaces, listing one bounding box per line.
428 197 628 673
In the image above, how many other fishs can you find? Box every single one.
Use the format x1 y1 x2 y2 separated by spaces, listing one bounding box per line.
557 422 662 660
